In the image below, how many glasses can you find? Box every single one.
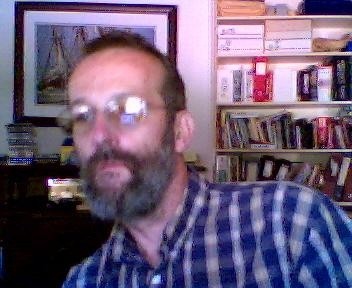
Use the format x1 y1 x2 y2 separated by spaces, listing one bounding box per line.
56 96 185 136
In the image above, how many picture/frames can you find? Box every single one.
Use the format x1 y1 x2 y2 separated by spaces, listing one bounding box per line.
11 1 178 127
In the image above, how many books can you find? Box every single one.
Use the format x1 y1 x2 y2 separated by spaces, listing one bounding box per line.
216 110 352 149
216 56 352 102
215 153 352 202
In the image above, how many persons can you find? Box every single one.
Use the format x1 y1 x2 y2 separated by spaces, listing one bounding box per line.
57 30 352 288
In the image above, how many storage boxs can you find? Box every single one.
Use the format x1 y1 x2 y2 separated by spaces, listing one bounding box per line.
304 0 352 15
216 38 264 55
217 0 267 16
264 37 314 52
217 25 265 39
264 19 312 38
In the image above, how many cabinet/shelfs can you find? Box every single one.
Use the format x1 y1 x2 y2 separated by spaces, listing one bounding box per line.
209 0 352 219
0 154 209 288
